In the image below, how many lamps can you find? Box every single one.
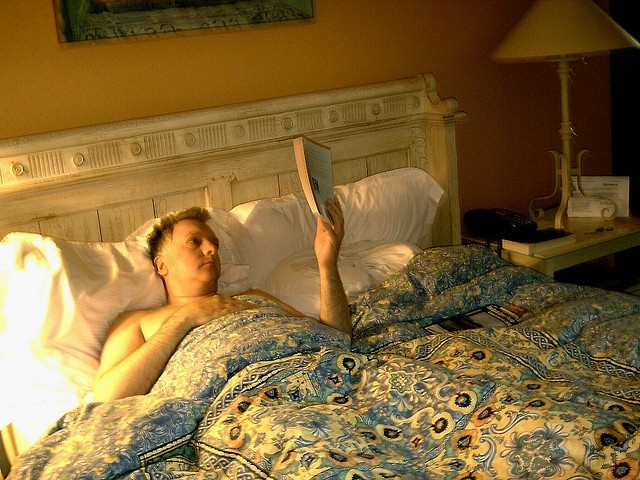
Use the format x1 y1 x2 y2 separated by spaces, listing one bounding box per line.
486 2 640 228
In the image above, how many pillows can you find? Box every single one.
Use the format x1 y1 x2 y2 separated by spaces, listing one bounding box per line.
226 166 442 316
1 211 258 447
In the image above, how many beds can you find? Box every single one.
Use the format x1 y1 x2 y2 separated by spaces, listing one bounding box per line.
1 72 639 479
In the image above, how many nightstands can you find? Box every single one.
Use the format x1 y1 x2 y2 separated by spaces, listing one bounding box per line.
463 207 640 289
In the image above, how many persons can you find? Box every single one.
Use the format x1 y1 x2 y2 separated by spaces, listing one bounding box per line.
93 196 354 403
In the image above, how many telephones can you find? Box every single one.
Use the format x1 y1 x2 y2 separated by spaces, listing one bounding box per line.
464 208 538 257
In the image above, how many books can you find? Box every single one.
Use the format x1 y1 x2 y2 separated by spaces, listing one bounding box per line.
501 226 576 257
291 132 338 225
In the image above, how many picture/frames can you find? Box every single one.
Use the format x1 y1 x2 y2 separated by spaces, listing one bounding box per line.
52 0 317 44
